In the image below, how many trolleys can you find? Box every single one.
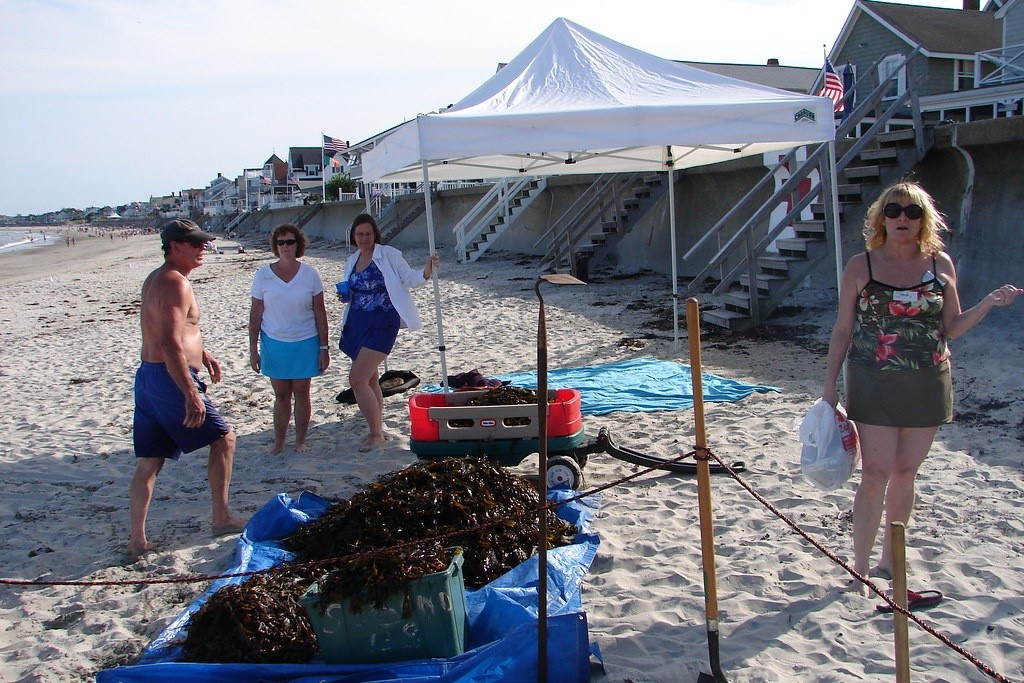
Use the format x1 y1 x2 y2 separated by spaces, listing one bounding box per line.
407 387 749 492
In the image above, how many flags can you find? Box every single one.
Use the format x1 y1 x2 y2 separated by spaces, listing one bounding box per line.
818 44 844 114
324 135 347 152
287 175 299 184
324 154 339 169
260 175 271 184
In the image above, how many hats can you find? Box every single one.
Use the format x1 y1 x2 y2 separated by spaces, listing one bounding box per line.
160 219 216 244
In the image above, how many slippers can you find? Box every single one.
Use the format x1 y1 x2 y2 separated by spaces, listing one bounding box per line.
876 590 942 613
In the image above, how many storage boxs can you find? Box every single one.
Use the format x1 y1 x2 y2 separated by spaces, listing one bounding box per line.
299 546 467 665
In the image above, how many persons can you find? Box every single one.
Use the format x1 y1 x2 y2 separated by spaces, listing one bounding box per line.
129 218 251 556
822 181 1024 600
29 223 159 248
248 223 331 457
335 213 440 454
203 230 236 252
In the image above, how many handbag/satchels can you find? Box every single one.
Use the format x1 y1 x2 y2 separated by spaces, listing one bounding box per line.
799 395 861 491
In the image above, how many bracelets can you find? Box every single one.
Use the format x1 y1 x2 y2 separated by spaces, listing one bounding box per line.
320 345 330 350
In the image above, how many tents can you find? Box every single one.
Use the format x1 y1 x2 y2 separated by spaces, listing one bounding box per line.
360 15 846 392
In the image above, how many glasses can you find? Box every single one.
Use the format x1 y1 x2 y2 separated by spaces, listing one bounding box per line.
276 239 298 246
881 202 924 220
